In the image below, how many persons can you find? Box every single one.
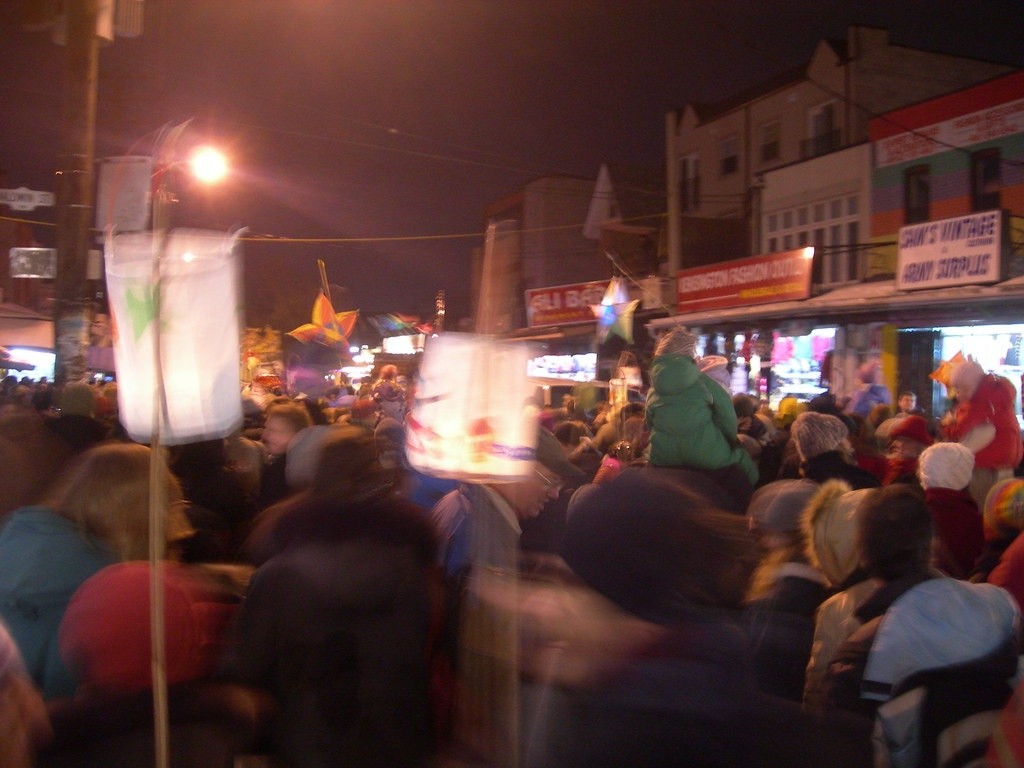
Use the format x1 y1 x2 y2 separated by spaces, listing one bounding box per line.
639 326 764 484
1 352 1024 768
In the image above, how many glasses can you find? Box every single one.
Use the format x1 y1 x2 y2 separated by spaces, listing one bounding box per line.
534 468 561 491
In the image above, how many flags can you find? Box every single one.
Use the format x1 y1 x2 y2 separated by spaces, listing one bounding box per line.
403 328 535 484
102 226 249 440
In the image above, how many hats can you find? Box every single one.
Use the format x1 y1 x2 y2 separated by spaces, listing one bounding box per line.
858 361 881 384
774 397 807 427
56 560 197 688
858 484 932 575
983 478 1024 541
875 415 933 446
790 411 848 461
697 356 731 386
951 354 984 400
917 442 975 490
748 479 819 531
537 425 587 477
655 326 697 357
